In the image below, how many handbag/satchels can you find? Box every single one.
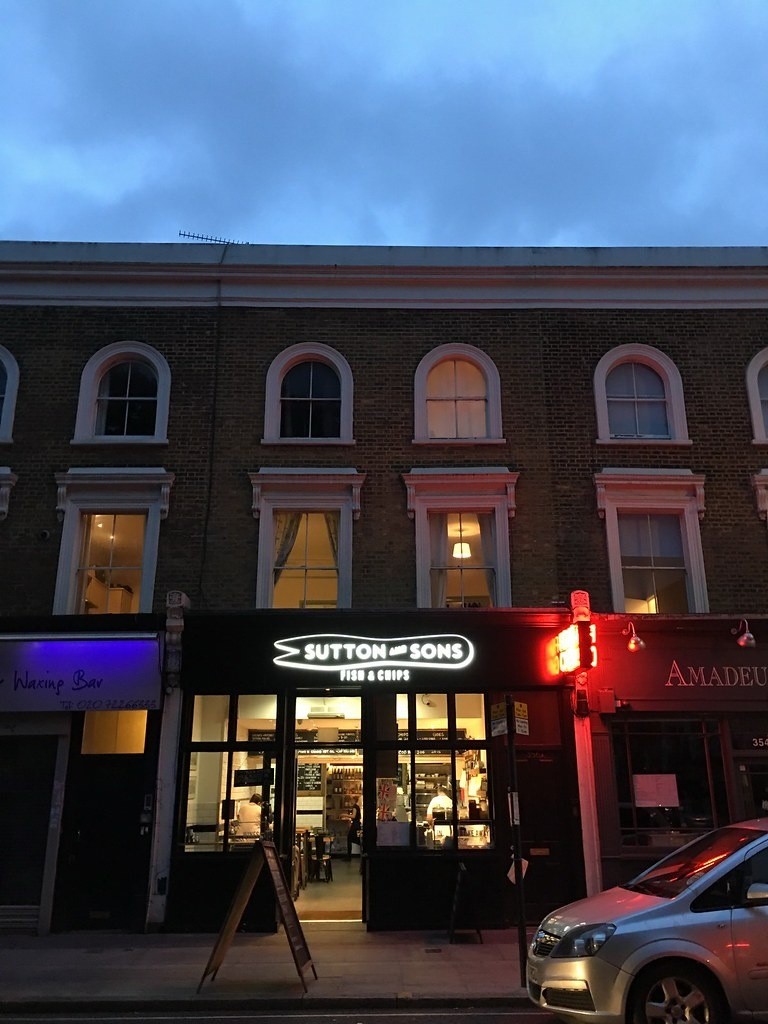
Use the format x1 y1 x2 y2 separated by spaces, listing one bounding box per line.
354 821 361 830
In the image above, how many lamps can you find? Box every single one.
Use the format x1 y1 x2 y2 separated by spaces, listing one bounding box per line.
453 529 472 559
622 622 646 653
732 618 756 648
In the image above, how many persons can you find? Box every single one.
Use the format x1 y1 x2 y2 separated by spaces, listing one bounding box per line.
426 784 453 837
340 797 362 861
235 793 263 835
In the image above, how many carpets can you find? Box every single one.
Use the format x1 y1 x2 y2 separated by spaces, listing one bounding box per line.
298 911 362 921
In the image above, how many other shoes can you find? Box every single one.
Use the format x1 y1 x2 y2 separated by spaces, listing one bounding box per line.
341 855 351 862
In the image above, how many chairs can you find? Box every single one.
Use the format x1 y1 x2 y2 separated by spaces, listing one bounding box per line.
308 835 333 883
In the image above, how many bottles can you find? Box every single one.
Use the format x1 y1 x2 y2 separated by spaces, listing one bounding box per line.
446 806 452 820
432 804 445 820
333 783 362 795
459 805 467 819
333 768 362 780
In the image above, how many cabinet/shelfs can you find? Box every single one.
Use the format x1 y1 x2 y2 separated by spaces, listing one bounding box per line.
88 574 132 613
326 765 478 821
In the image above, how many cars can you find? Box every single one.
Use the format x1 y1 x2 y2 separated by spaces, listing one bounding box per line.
524 817 768 1024
